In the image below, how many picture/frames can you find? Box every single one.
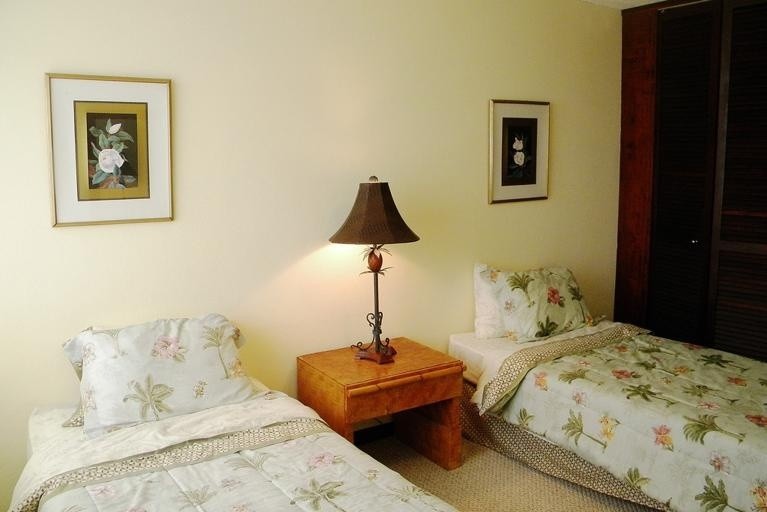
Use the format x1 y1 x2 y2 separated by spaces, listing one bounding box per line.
44 71 174 228
489 99 551 205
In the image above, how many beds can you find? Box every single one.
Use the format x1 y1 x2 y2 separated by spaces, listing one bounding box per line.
27 378 459 512
450 315 767 512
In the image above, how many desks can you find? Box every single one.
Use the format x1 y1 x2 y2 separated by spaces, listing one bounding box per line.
297 335 461 471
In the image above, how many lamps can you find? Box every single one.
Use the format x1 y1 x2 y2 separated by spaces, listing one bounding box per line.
329 175 420 357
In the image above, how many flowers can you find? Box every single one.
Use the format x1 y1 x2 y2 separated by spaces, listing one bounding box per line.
88 116 137 190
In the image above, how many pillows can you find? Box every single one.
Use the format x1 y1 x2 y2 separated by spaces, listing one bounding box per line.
474 263 509 339
494 265 594 345
62 313 255 441
